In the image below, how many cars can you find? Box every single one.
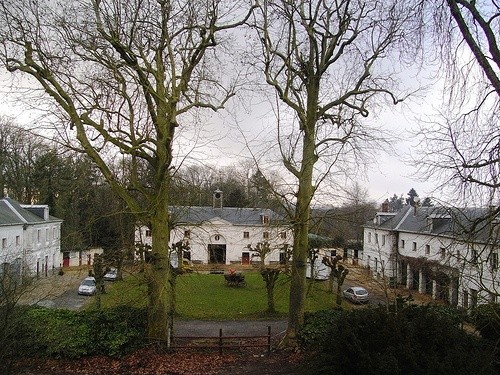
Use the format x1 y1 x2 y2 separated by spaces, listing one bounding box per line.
104 268 117 281
343 287 369 303
78 277 96 296
